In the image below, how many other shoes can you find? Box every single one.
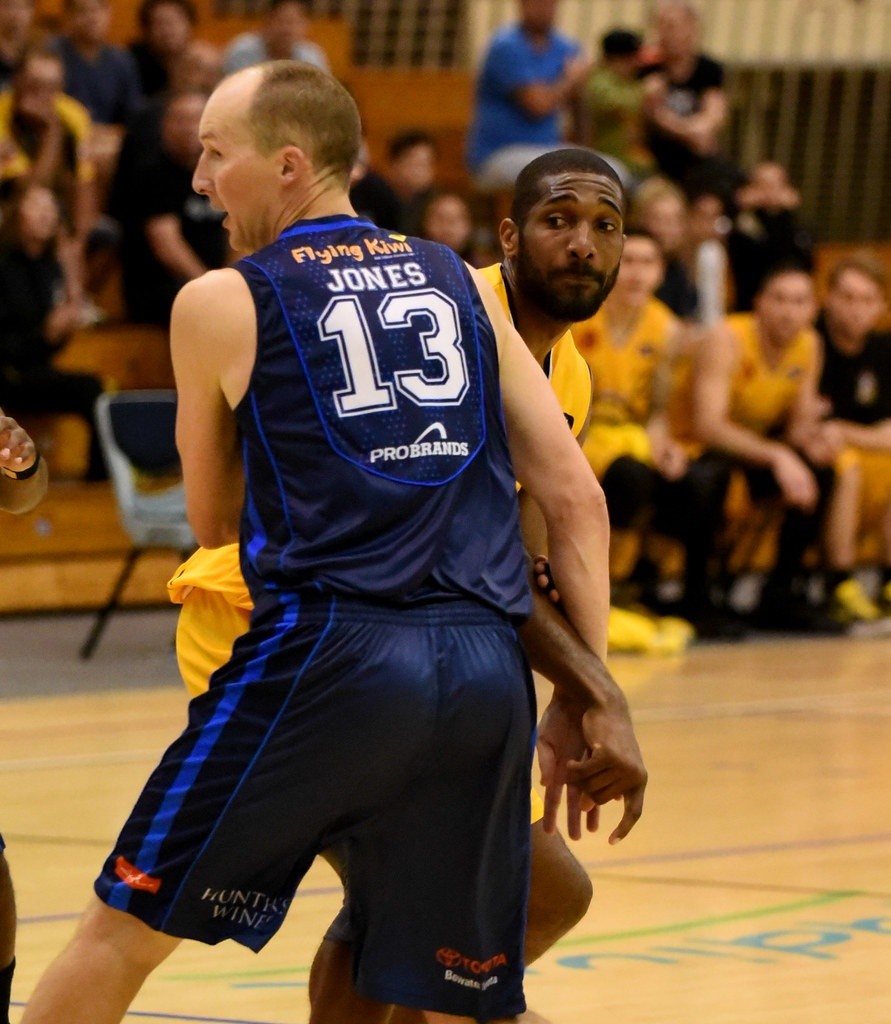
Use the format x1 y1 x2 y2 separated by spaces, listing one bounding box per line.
680 589 742 641
759 588 846 634
75 460 111 487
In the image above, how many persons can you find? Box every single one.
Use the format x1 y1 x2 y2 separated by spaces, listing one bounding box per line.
467 0 810 325
346 128 475 268
545 230 891 630
165 147 648 1022
0 0 329 479
18 58 609 1024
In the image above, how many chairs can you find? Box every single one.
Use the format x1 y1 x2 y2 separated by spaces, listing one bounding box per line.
80 388 199 658
